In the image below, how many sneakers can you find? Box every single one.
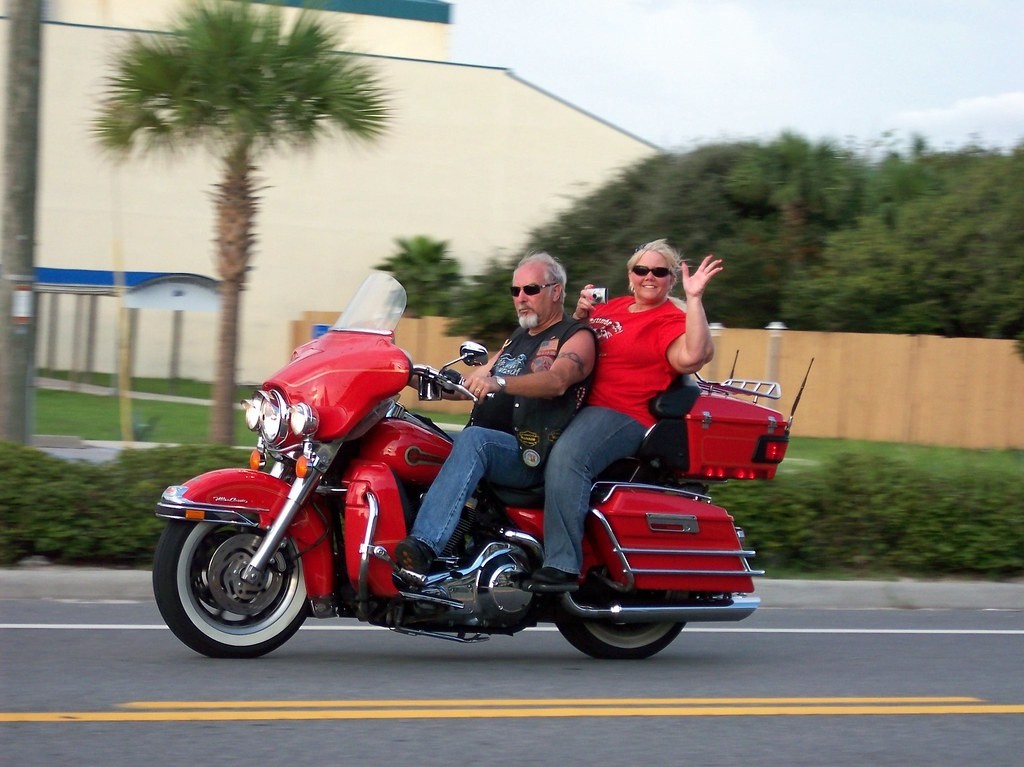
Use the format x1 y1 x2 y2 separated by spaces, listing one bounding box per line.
395 536 434 593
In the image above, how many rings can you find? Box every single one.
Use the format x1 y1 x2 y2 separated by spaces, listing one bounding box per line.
475 388 481 394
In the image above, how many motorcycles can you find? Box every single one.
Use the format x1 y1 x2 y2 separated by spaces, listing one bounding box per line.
151 271 792 659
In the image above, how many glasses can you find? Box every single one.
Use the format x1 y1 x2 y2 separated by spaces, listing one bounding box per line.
510 282 557 297
632 265 673 278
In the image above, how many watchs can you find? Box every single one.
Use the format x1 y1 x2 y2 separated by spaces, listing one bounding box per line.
492 376 506 395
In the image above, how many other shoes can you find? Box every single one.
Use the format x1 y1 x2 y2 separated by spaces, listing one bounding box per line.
531 567 580 583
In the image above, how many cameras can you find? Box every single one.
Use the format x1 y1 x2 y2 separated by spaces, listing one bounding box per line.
586 288 609 305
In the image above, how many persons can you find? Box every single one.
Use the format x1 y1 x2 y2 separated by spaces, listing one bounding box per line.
532 236 725 585
394 254 599 575
